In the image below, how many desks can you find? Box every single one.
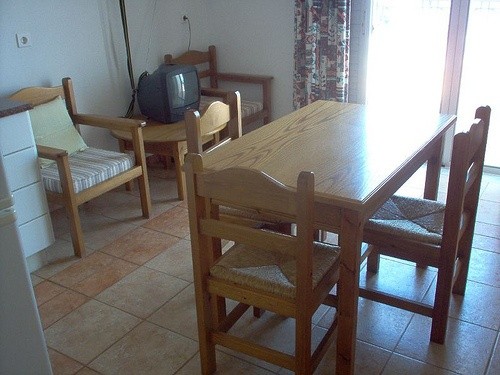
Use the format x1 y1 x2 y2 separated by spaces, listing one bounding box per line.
203 100 458 375
110 115 221 202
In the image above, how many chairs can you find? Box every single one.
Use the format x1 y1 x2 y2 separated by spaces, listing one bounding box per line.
359 105 492 345
182 152 344 375
164 46 274 139
184 90 295 238
8 78 151 258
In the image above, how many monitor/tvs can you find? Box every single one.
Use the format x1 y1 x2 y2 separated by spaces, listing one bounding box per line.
137 63 201 125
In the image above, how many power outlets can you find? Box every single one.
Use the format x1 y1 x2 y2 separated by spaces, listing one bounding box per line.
182 15 187 24
15 33 32 48
